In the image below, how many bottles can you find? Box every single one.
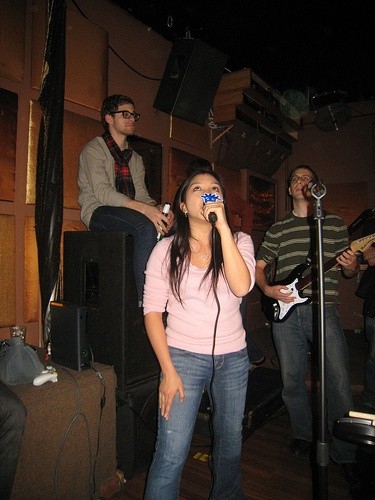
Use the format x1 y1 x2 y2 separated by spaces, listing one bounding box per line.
157 202 171 241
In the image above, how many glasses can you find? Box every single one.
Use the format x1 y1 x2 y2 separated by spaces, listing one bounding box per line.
288 175 312 182
110 111 140 121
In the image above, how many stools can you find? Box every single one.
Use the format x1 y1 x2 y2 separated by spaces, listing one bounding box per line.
332 411 375 500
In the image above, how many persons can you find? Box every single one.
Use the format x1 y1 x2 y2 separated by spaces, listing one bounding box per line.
346 207 375 416
76 94 176 310
0 379 27 500
141 168 257 500
253 165 362 484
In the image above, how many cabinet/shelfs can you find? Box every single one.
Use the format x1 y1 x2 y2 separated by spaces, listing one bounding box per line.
213 68 303 150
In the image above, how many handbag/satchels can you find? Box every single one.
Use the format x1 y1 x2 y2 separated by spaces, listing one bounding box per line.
0 337 44 386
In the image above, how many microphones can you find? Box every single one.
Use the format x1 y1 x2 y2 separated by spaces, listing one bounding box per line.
201 193 222 224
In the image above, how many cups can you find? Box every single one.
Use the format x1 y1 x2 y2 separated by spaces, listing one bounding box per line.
10 325 25 342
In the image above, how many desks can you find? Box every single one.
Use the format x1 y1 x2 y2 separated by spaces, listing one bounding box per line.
6 346 117 500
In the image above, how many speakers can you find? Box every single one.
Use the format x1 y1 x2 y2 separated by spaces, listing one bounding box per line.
60 230 169 480
153 37 227 127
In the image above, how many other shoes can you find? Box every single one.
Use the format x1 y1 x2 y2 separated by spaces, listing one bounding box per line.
361 399 375 408
339 462 358 484
289 438 309 459
